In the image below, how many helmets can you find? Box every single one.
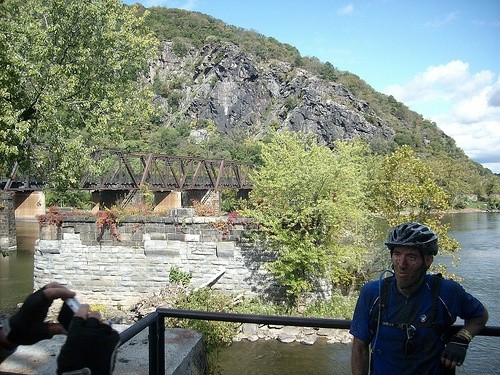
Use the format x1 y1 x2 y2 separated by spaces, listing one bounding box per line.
384 221 440 253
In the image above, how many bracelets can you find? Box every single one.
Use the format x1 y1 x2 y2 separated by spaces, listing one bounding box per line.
457 328 474 341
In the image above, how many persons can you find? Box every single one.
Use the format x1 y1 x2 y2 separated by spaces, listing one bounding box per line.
0 282 120 375
348 222 489 375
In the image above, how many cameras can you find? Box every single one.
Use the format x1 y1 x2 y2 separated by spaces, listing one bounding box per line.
56 298 80 334
